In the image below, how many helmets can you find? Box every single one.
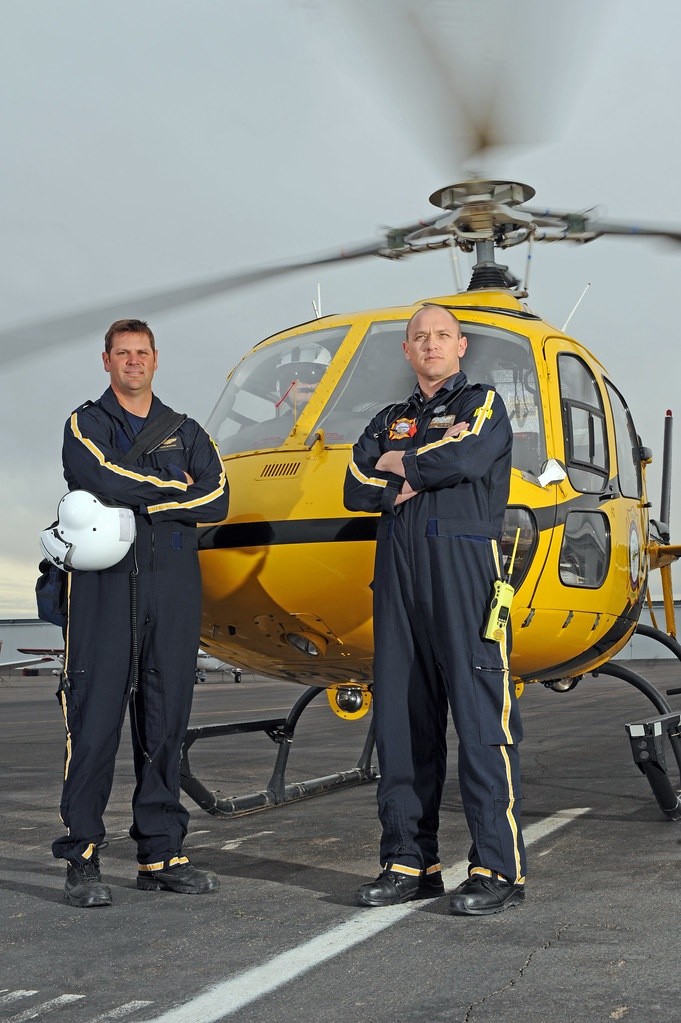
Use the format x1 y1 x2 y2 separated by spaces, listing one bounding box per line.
39 487 136 570
274 342 333 407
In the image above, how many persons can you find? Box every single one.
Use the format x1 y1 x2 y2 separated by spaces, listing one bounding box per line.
224 343 331 449
343 305 526 914
50 320 230 907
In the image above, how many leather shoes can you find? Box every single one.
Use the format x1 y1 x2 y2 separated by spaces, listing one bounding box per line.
449 876 527 914
136 862 219 894
356 869 445 907
64 842 112 908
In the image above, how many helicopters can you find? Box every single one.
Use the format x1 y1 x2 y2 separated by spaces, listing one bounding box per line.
2 0 681 825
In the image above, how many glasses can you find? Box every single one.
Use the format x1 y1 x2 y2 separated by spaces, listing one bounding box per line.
282 366 322 383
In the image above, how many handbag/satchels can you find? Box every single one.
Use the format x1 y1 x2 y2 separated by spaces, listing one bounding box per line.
34 560 67 627
